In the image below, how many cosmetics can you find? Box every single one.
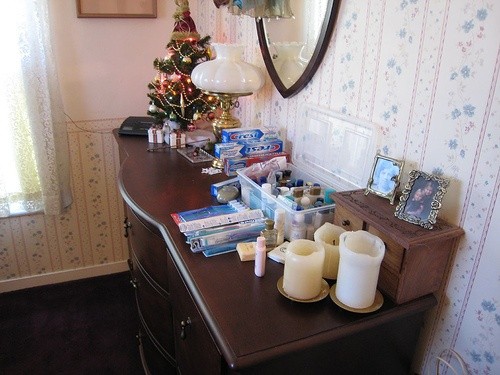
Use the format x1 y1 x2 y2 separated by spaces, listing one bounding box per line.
243 170 336 239
148 124 185 148
255 209 285 277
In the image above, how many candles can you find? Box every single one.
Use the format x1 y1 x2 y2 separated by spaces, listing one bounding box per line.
283 222 385 308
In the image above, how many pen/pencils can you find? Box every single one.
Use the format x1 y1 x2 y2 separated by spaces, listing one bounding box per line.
194 146 200 156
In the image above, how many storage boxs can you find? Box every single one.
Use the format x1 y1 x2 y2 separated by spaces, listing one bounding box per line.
216 102 380 241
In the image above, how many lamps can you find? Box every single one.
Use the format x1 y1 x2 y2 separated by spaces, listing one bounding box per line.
192 44 264 143
215 0 297 23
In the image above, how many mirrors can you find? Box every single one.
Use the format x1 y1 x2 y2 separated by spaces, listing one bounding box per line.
257 0 338 97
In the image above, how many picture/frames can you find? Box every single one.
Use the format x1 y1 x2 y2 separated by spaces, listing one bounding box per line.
76 0 158 18
395 169 449 229
365 154 404 206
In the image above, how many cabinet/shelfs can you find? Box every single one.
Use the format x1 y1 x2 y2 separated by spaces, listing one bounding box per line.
113 128 462 375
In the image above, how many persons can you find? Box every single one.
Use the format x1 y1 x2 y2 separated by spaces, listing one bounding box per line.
405 180 435 219
376 162 398 193
